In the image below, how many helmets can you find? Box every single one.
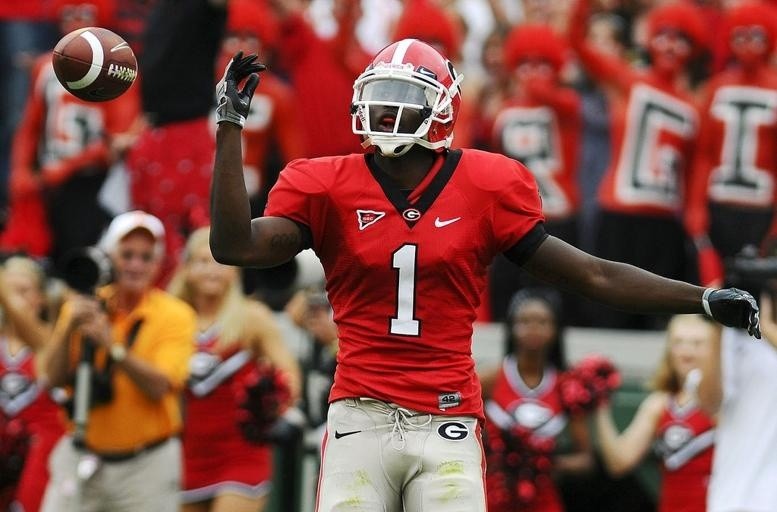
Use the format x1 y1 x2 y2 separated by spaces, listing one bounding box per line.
349 39 461 158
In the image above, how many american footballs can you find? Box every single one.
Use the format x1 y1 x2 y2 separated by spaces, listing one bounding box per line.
52 27 138 102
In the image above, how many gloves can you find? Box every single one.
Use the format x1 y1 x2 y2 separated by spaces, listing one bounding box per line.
215 50 267 129
701 286 763 339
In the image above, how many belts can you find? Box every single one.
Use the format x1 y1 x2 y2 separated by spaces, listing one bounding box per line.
72 437 166 462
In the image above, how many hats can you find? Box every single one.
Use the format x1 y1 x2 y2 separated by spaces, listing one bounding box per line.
111 209 165 242
